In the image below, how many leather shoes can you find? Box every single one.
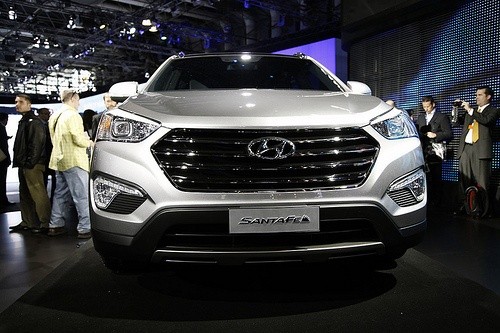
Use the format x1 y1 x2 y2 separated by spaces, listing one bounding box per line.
448 204 467 215
0 201 16 207
9 224 36 230
472 211 496 220
48 227 65 235
32 227 47 232
78 232 92 238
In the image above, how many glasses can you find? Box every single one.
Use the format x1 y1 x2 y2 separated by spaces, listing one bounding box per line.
72 90 76 98
41 112 50 115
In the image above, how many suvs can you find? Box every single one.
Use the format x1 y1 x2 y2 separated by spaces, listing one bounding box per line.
82 50 435 274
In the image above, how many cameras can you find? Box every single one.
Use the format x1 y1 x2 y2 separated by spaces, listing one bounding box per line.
452 102 462 107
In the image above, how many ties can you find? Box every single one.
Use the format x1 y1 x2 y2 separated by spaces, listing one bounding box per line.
473 109 481 142
428 113 431 117
